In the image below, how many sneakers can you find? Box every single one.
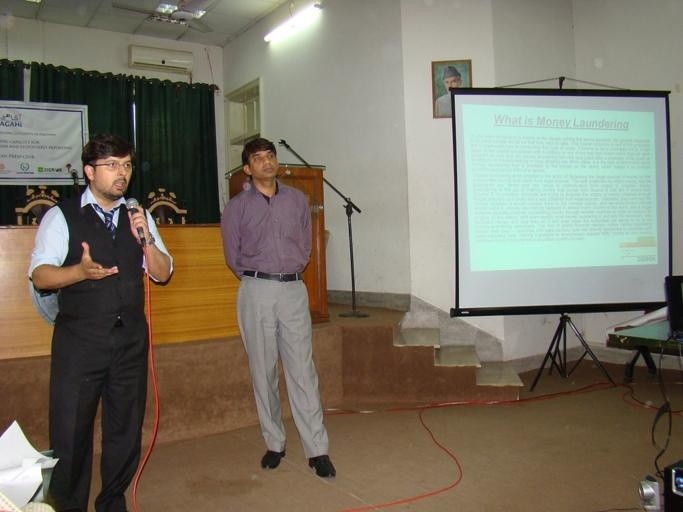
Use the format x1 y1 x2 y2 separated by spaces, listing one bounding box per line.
262 449 285 469
309 456 336 478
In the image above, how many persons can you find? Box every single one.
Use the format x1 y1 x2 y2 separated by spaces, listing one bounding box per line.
435 65 463 116
219 135 338 479
28 132 174 512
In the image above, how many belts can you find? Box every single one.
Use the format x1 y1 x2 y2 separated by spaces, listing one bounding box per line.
243 270 304 281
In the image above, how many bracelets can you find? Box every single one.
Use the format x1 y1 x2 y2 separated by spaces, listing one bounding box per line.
138 233 156 246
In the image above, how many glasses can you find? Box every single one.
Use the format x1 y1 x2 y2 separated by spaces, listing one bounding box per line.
91 161 134 169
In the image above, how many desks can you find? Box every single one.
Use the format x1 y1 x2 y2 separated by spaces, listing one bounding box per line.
603 316 683 385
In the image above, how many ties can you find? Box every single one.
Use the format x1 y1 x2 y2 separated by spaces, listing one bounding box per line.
93 203 120 239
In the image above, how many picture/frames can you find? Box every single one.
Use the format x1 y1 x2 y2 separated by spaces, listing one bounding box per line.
429 58 473 119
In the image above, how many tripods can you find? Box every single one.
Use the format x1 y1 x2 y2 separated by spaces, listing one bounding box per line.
528 315 620 390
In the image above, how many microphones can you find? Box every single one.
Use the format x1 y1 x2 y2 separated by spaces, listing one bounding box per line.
70 168 80 185
126 199 148 246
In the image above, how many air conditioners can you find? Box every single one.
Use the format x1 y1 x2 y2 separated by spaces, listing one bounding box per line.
126 42 195 75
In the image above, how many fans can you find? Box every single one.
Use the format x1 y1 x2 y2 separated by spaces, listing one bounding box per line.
110 1 223 36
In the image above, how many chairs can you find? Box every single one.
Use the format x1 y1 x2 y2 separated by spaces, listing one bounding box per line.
14 184 64 227
140 187 188 224
